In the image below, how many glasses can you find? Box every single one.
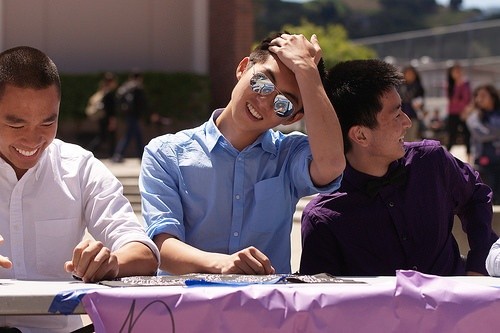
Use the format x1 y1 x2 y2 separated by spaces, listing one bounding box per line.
250 64 295 117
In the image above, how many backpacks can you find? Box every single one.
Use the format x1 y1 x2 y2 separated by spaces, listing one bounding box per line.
85 88 110 120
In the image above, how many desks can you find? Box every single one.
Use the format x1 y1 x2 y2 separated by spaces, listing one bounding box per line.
0 270 500 333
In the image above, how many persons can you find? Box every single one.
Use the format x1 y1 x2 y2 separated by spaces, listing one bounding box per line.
139 30 347 275
300 60 499 278
86 71 168 163
385 57 499 207
0 45 160 333
